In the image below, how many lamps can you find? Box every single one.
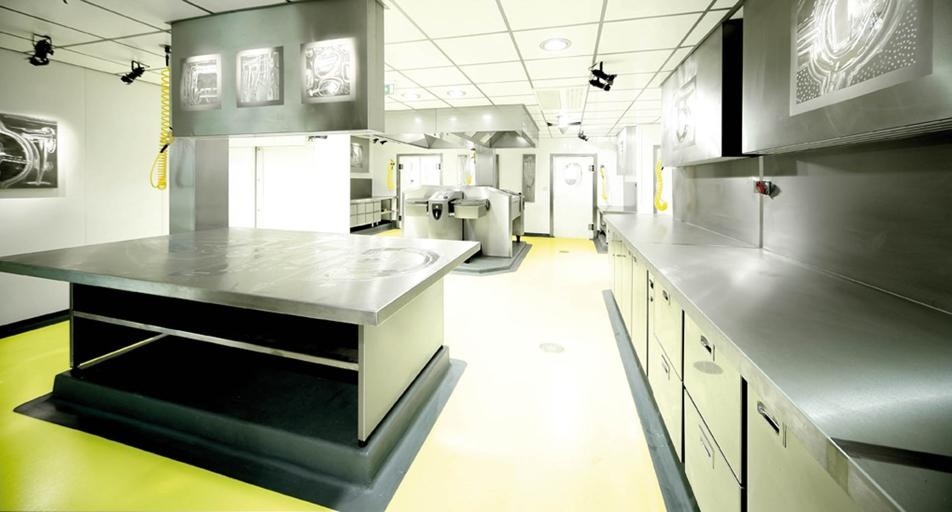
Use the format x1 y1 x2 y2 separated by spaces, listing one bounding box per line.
578 131 586 141
373 138 387 146
589 61 617 91
121 59 147 84
26 33 55 67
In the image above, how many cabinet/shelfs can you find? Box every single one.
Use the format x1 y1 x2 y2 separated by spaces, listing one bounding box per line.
746 376 863 511
350 196 399 228
682 309 748 512
606 223 649 379
648 268 685 469
